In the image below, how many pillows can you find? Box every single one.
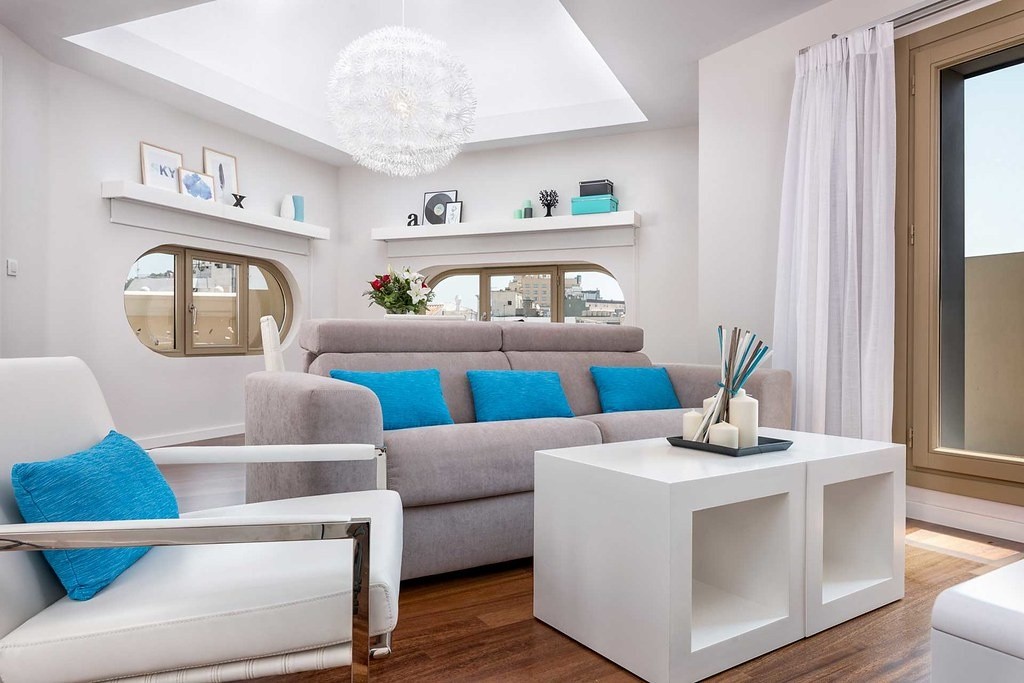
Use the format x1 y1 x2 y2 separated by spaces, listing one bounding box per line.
10 431 181 602
330 369 455 429
466 369 576 422
590 366 681 413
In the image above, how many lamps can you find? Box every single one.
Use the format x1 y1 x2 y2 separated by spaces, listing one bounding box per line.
324 0 479 178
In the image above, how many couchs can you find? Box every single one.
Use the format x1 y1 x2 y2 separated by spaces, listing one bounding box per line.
243 314 793 583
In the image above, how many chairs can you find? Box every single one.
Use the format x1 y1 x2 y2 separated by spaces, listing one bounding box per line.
1 356 406 682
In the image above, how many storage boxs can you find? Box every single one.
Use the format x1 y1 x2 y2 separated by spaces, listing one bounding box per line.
572 179 619 215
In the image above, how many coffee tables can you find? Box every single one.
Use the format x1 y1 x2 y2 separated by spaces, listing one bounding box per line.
534 425 905 683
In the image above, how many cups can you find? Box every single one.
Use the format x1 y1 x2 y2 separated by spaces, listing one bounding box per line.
514 209 522 219
292 195 304 222
523 207 533 218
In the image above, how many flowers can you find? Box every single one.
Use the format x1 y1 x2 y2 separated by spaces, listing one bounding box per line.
364 265 435 315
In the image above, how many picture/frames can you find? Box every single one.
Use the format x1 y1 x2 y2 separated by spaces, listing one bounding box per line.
445 201 463 224
421 190 458 225
202 146 238 207
140 141 184 193
178 167 216 202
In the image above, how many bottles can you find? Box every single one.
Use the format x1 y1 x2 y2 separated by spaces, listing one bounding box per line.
280 194 295 220
